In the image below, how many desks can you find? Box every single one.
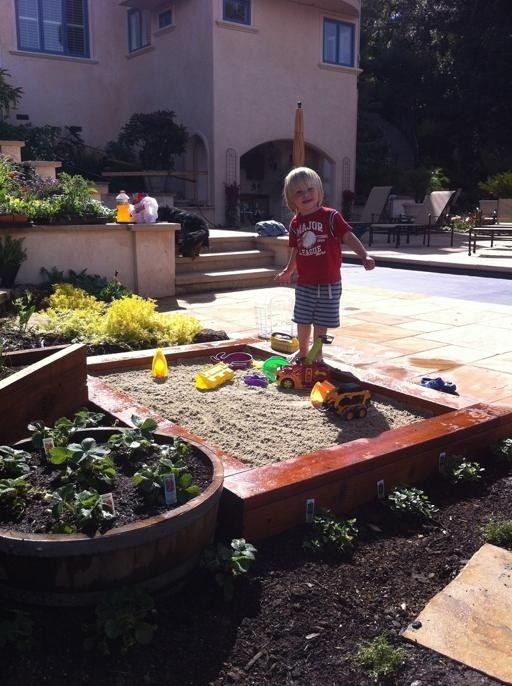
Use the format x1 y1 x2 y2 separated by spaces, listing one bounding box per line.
241 194 268 210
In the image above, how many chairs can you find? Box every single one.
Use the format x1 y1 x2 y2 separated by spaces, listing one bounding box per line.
346 186 512 255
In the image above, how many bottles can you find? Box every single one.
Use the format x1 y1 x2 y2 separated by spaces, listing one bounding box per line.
115 189 132 222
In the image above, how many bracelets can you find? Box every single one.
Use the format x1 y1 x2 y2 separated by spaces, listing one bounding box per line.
283 269 291 276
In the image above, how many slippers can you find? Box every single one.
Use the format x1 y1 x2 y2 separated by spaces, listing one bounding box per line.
421 378 458 396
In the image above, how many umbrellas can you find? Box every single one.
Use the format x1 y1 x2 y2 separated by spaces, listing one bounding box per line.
290 100 305 168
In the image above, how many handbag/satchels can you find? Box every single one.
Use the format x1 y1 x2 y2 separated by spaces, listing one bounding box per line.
255 220 289 237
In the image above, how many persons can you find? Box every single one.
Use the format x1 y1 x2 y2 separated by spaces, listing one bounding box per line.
274 165 378 365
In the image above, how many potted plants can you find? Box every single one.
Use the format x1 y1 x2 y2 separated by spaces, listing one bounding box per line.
0 171 113 228
0 233 28 287
